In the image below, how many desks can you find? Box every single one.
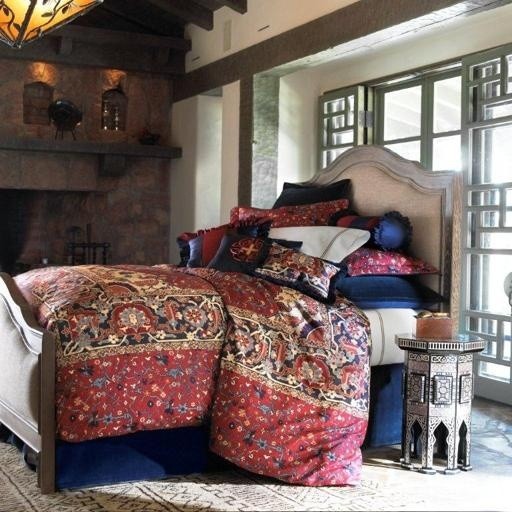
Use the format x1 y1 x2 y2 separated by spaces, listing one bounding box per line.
395 334 485 475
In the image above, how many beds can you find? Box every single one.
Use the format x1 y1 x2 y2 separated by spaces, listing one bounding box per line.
1 140 469 494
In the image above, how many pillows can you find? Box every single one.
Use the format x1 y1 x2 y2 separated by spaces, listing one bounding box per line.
175 180 449 308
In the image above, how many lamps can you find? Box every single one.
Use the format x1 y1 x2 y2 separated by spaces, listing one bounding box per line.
0 0 105 50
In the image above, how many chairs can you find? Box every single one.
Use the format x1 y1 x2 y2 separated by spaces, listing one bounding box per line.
66 243 111 265
